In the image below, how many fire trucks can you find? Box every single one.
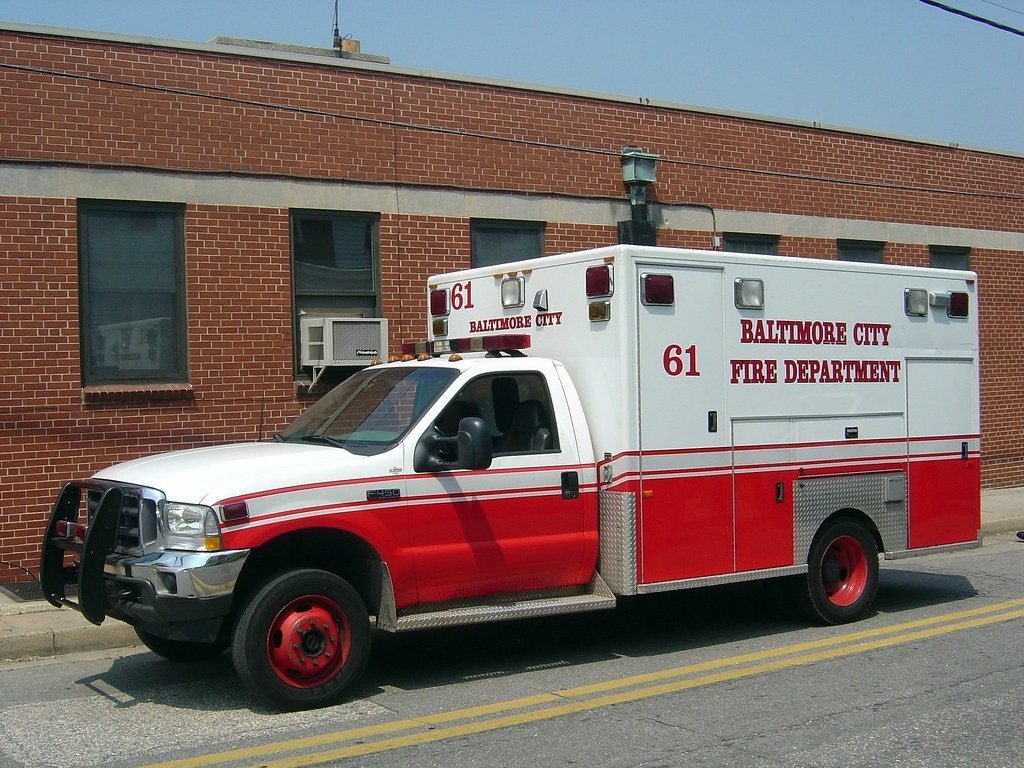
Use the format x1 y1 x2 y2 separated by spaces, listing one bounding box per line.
35 242 985 719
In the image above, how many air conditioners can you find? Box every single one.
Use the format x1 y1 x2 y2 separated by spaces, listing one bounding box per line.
300 317 389 367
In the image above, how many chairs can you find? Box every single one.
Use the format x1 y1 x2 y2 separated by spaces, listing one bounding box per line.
497 398 553 452
439 401 482 438
